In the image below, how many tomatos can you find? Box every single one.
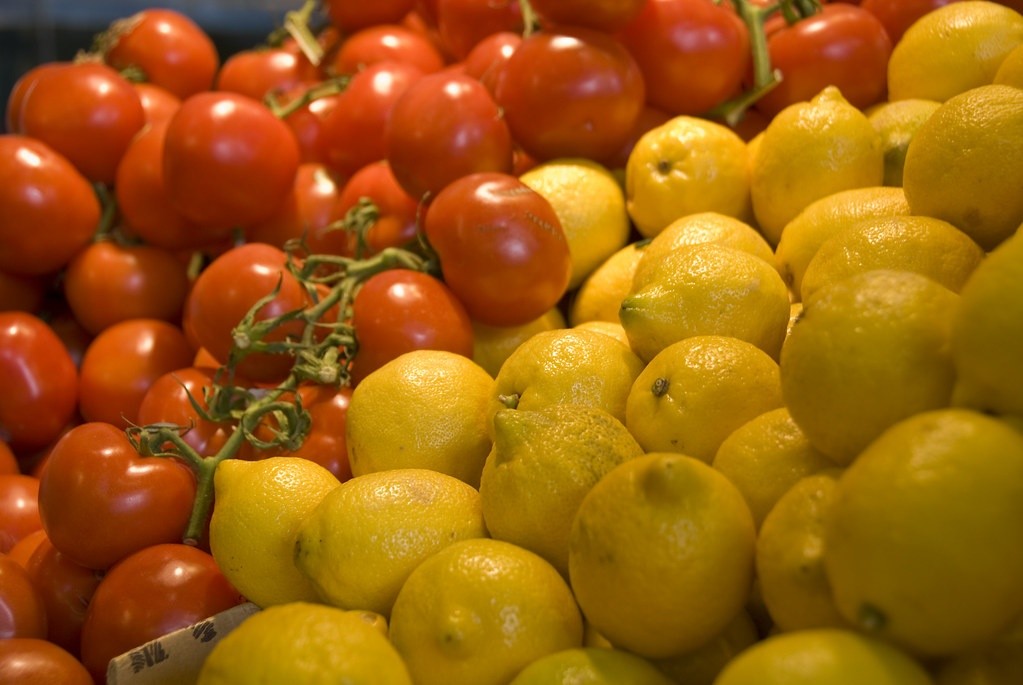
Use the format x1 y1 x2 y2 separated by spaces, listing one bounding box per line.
0 0 930 685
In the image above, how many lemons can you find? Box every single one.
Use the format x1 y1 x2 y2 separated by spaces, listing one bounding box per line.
205 0 1023 685
